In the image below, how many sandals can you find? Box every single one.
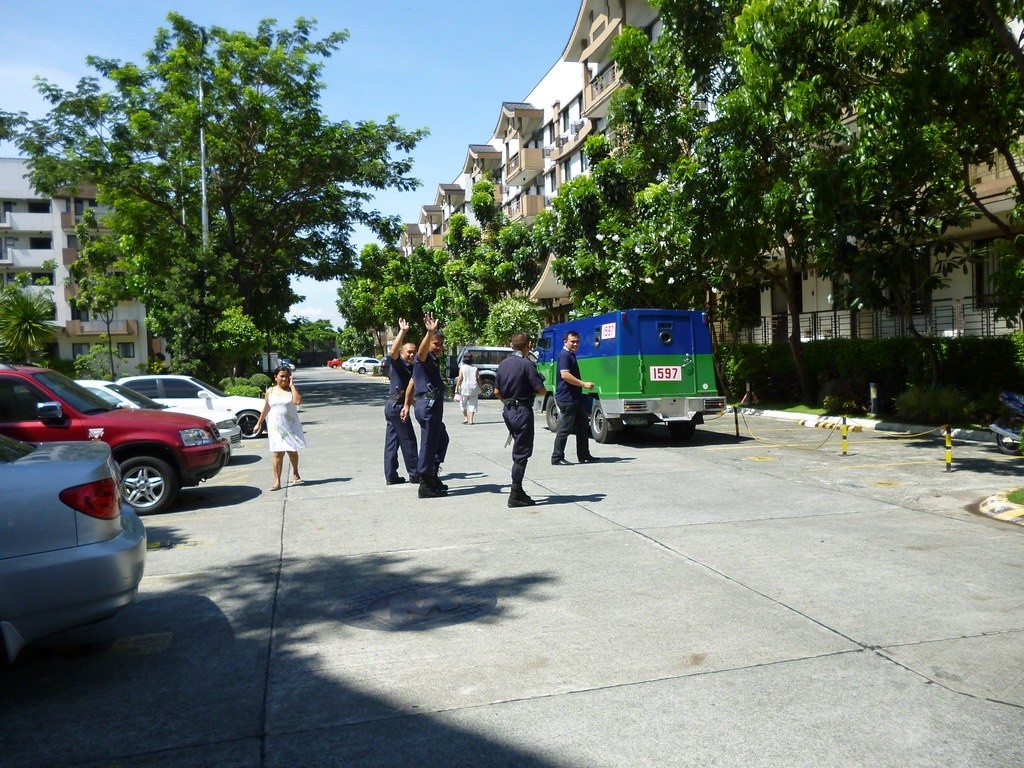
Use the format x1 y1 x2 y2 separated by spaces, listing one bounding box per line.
462 420 474 424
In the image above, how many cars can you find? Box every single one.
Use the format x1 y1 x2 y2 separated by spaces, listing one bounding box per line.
71 376 242 448
0 428 146 667
327 356 382 373
1 362 228 514
116 377 276 438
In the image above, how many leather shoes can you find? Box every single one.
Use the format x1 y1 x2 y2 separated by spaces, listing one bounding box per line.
551 457 574 465
579 456 601 463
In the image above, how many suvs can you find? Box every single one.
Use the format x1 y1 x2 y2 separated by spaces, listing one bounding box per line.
456 341 528 397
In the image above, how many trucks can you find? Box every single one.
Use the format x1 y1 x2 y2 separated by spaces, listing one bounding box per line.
532 309 728 440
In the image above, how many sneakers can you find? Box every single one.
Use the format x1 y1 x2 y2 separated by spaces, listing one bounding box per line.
418 479 449 496
508 489 535 507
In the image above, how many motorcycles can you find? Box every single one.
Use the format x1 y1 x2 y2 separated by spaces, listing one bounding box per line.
988 388 1024 452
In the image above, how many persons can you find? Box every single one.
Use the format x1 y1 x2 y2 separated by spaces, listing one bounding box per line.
455 353 485 424
254 364 307 491
551 331 602 466
384 317 417 485
413 310 450 499
494 332 547 508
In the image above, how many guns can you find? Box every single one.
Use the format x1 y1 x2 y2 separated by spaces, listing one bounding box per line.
425 383 440 409
391 384 404 410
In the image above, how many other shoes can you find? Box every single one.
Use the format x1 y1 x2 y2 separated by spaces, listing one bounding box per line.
387 476 406 485
410 474 419 483
294 476 300 484
270 486 281 490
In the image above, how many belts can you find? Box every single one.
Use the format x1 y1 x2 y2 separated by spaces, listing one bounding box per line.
503 400 530 407
